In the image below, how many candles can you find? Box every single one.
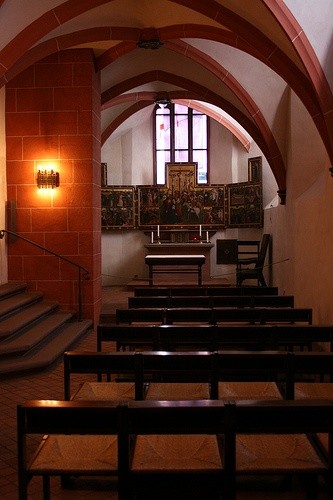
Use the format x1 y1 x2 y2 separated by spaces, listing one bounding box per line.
206 231 208 242
200 225 201 236
158 225 160 236
152 232 153 243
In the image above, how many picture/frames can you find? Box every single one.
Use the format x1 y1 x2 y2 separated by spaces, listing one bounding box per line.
100 157 263 229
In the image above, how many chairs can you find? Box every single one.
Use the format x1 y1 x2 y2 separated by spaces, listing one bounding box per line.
237 233 270 286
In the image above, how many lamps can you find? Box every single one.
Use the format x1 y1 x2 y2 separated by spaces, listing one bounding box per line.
34 163 59 196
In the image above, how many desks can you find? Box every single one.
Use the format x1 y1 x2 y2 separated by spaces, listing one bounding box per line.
146 243 215 280
146 255 205 286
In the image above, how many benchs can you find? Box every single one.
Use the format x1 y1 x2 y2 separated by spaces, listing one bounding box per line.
17 287 333 500
